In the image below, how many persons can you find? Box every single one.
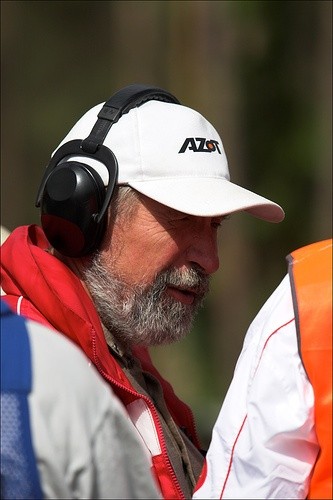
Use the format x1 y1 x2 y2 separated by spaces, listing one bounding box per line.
0 84 287 499
191 237 333 499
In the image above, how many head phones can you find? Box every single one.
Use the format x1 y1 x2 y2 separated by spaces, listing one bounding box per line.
36 82 178 259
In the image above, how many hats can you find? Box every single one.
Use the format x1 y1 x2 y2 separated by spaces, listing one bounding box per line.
51 98 285 223
